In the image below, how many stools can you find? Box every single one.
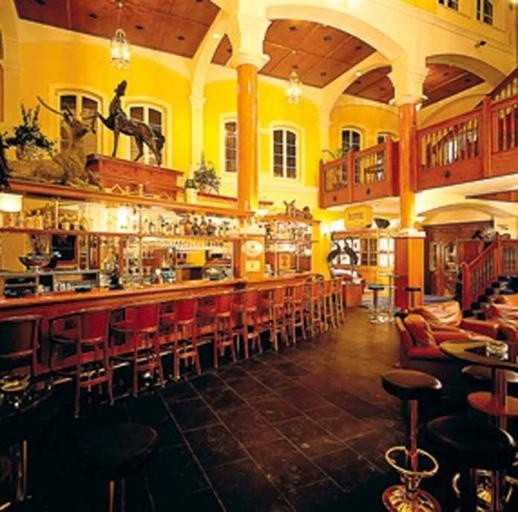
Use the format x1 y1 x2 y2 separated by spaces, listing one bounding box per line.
380 369 443 512
85 423 160 511
368 285 388 324
425 414 515 512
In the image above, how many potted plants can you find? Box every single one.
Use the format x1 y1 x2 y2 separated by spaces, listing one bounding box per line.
192 153 221 196
1 102 57 161
322 148 348 189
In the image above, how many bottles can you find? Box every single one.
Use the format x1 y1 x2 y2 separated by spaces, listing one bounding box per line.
4 197 307 290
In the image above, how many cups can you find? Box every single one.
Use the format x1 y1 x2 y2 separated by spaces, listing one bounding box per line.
485 340 509 361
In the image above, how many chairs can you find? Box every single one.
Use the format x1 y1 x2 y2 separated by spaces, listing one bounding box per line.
394 287 518 375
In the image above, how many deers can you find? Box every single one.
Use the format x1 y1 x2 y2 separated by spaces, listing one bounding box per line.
7 96 105 190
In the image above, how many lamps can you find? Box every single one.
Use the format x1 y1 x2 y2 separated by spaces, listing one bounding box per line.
286 48 304 103
111 0 133 69
